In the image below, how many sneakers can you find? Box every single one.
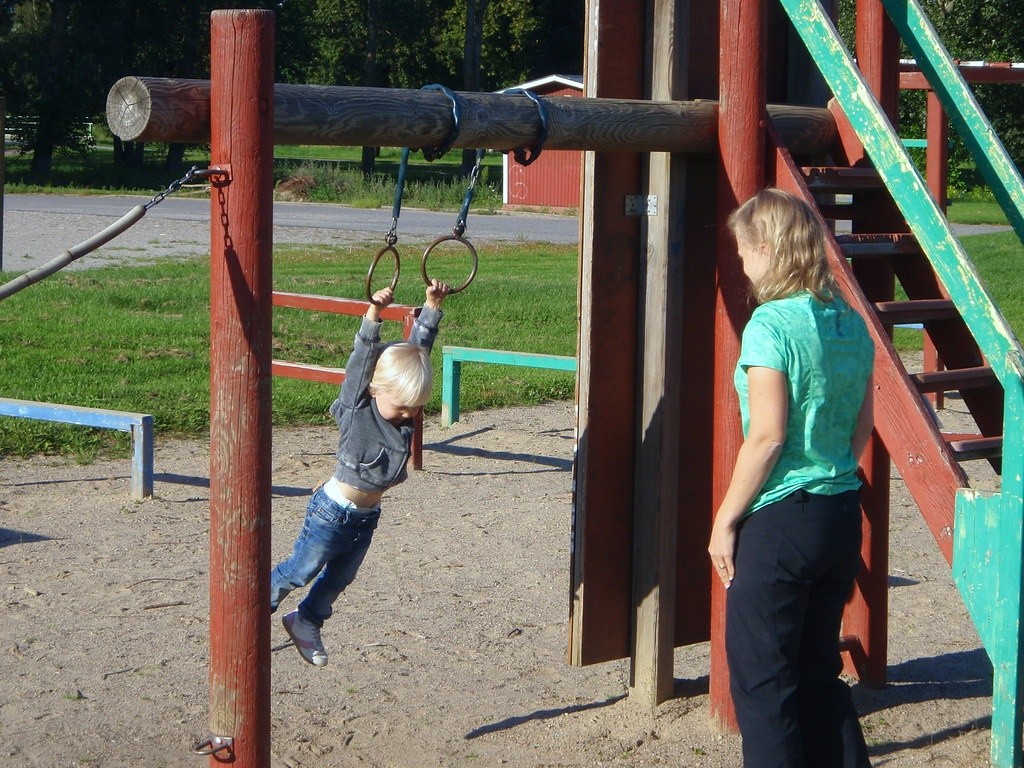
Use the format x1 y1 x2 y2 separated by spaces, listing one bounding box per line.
282 609 328 667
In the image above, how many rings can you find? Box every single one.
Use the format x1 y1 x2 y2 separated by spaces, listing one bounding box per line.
718 564 726 569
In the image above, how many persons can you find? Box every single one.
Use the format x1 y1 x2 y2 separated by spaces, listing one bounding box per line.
260 280 451 669
707 190 877 768
